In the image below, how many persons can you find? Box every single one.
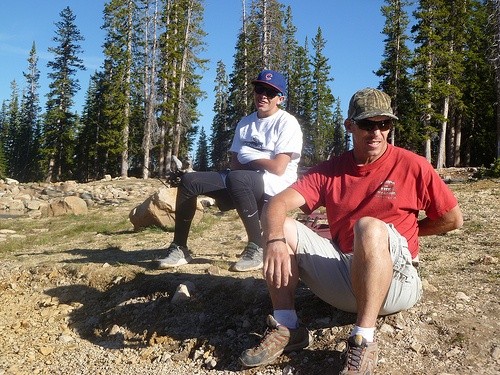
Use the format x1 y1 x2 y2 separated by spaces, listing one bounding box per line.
153 68 307 273
233 85 465 375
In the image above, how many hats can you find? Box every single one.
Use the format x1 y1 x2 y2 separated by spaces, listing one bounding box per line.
251 70 287 98
345 86 401 123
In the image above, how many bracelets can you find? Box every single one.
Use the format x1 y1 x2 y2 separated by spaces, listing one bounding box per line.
265 237 288 246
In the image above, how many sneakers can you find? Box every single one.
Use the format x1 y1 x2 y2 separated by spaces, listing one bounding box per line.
336 335 379 375
237 314 310 367
233 240 266 273
158 244 194 270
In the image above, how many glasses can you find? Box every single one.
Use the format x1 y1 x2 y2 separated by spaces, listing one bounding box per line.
254 84 283 100
350 115 395 132
